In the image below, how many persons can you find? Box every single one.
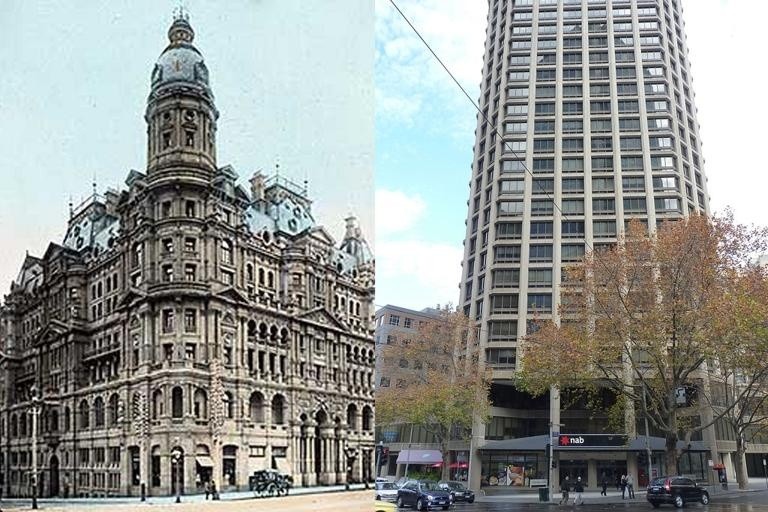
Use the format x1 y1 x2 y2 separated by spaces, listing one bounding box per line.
62 471 71 498
204 477 211 500
601 475 608 496
625 471 635 498
558 474 571 505
211 479 220 500
573 475 584 504
620 473 628 499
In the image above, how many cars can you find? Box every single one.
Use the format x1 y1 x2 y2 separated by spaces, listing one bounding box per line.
374 476 475 511
249 469 293 495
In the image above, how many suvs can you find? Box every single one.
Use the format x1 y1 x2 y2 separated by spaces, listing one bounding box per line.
646 475 710 508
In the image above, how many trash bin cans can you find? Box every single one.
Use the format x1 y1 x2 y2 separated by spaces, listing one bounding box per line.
539 488 549 502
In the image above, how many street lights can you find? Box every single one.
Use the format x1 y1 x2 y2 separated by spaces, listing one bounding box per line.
25 383 42 507
170 450 185 504
761 457 768 486
